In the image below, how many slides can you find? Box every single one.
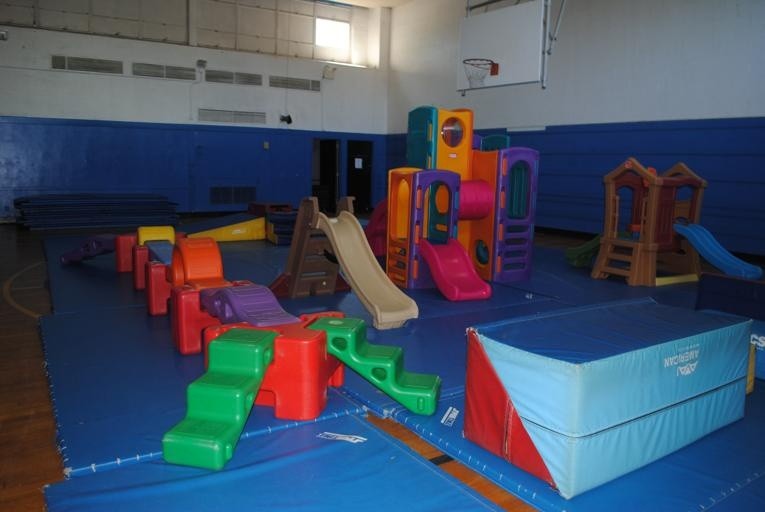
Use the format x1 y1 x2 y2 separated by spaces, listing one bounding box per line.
420 238 493 302
315 210 420 329
670 222 762 279
